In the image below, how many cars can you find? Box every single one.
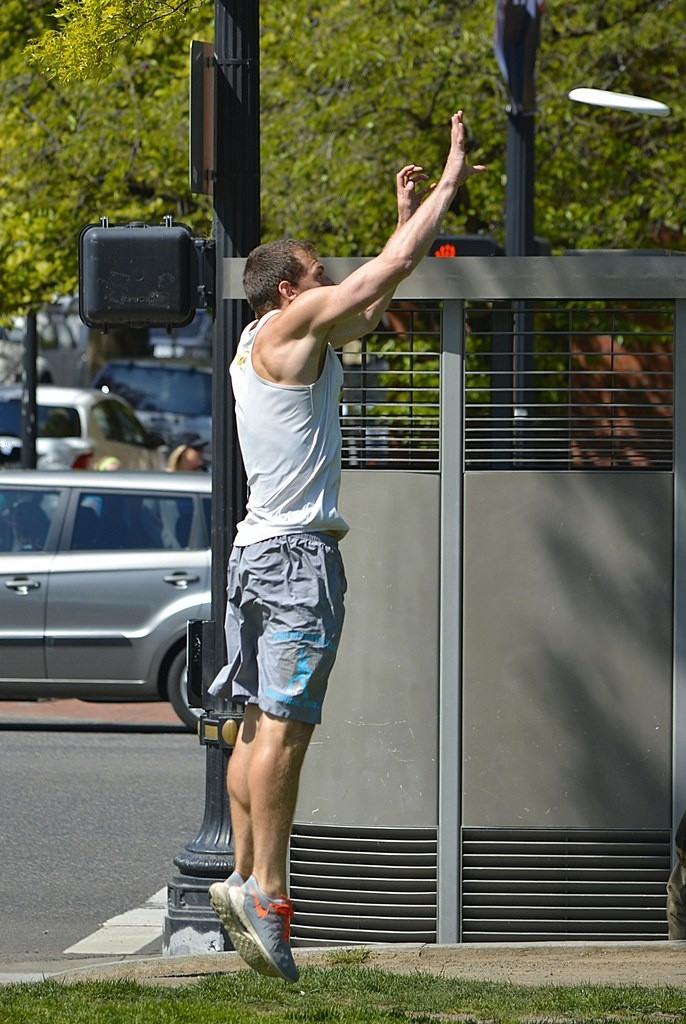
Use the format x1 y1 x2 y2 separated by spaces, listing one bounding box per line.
0 288 212 472
0 468 213 733
0 381 171 474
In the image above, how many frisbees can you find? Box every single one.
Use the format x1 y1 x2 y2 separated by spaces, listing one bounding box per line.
568 87 670 117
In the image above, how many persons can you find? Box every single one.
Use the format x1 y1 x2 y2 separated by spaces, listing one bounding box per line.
80 457 130 547
161 445 211 551
202 106 474 985
10 503 99 552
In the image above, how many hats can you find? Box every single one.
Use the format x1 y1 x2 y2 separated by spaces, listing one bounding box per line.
166 432 209 471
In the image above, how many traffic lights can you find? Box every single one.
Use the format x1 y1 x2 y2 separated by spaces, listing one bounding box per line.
433 242 457 258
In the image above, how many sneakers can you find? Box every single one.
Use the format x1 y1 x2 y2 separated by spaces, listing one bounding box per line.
207 870 301 986
